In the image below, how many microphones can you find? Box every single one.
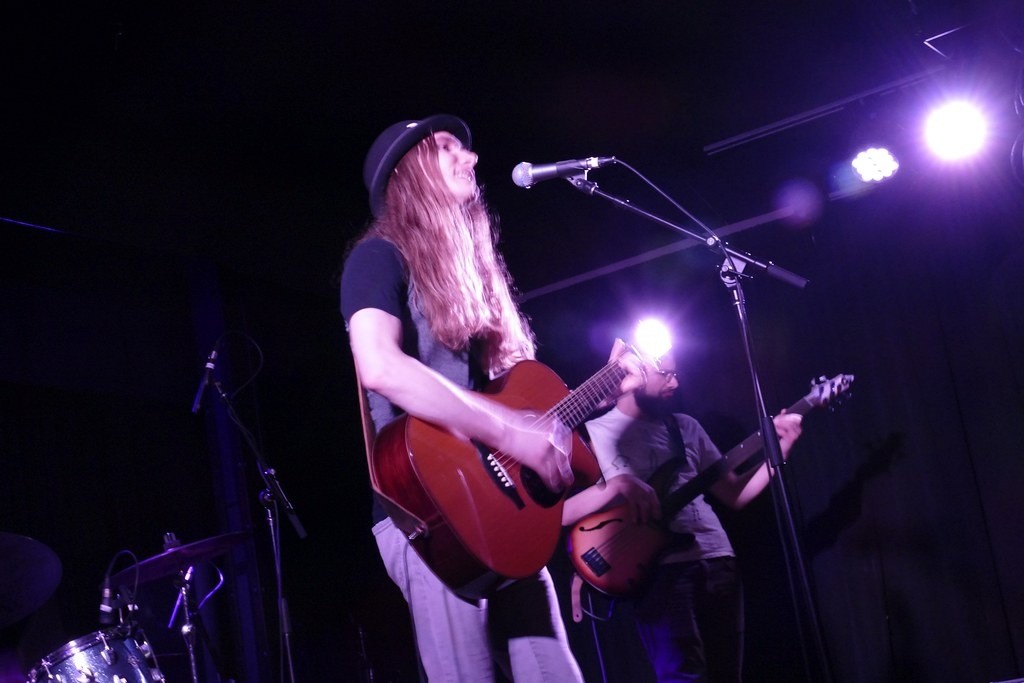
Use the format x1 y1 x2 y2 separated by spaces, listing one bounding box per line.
512 157 616 189
193 349 217 414
168 566 194 629
100 575 111 623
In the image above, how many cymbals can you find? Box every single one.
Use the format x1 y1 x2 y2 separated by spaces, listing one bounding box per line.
0 530 62 631
99 527 251 599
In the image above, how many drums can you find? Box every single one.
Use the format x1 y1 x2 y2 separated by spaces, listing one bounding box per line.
24 618 166 683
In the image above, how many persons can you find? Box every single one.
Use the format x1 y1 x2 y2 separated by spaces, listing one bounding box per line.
336 114 647 683
563 320 803 683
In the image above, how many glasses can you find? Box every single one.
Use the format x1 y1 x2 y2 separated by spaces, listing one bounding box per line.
654 368 682 378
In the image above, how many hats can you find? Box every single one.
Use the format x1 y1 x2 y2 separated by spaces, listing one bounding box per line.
363 113 474 220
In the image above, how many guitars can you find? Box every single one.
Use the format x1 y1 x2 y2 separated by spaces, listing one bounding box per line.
368 323 664 605
566 364 862 602
